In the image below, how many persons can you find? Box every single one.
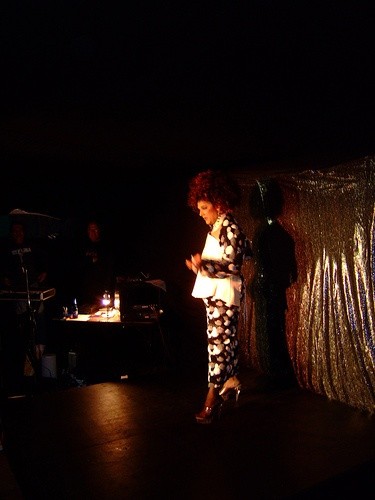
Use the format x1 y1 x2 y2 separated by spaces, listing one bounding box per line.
0 218 50 385
181 171 255 423
76 220 117 371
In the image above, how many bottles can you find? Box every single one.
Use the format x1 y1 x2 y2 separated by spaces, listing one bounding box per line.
72 298 78 318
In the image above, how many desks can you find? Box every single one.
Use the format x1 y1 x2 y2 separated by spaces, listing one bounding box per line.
64 303 172 384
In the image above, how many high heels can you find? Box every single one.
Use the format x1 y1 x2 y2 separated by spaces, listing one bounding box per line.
195 400 223 425
219 375 241 402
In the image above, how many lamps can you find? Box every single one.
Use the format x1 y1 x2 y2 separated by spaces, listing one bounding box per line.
113 292 120 311
101 290 112 318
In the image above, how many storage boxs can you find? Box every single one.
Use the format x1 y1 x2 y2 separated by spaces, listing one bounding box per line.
125 275 161 308
